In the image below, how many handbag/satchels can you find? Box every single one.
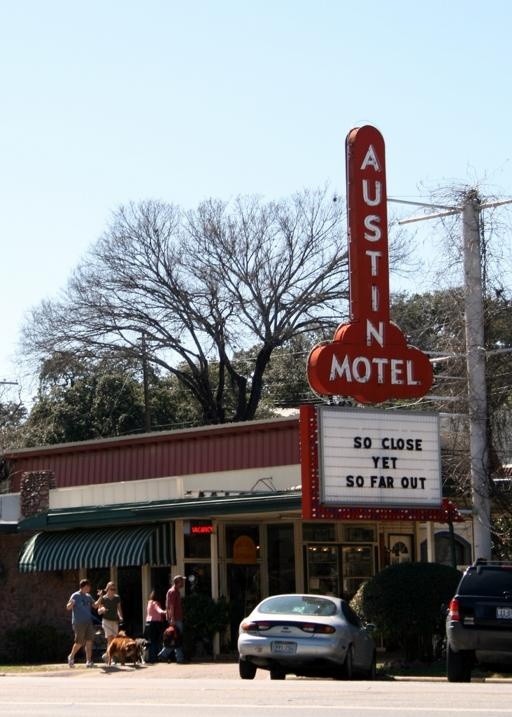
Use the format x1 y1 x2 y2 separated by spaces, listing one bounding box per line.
98 606 108 616
163 627 181 648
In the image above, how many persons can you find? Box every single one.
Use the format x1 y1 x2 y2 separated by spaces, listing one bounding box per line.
157 574 190 662
98 580 124 663
65 579 104 668
145 589 168 662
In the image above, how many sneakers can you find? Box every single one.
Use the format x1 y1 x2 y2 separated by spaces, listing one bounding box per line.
87 661 92 668
68 655 75 668
102 653 108 663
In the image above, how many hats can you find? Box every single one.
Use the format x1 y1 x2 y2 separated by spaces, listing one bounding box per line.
173 576 187 582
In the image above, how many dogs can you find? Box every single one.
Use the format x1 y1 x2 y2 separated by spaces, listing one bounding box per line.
106 634 151 666
135 638 151 664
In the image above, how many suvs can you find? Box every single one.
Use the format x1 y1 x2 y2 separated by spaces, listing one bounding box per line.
443 557 512 683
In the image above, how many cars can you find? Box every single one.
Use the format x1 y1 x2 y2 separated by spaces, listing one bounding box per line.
235 594 376 683
